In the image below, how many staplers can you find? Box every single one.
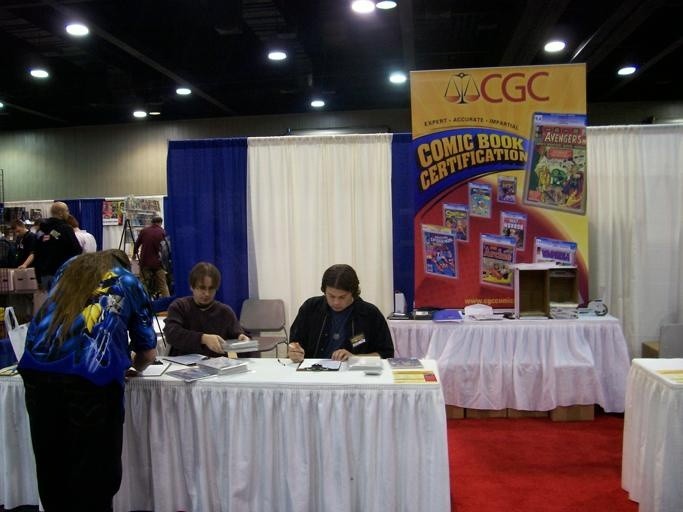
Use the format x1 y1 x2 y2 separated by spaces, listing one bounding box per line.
504 312 516 319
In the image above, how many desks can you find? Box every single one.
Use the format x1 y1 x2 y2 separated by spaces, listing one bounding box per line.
385 311 630 421
0 353 453 511
618 356 682 511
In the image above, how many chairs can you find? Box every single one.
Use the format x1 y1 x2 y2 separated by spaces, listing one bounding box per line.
658 324 682 357
236 299 290 358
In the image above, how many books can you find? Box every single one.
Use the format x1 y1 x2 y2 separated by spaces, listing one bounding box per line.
221 339 259 352
129 354 248 382
388 357 432 373
348 355 382 370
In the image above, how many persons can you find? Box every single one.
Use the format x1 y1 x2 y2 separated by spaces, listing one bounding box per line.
162 261 251 358
0 201 97 292
16 249 157 512
132 216 170 298
288 264 394 362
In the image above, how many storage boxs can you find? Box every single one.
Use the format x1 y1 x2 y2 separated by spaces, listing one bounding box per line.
13 267 39 289
465 407 508 420
444 404 464 419
0 267 14 291
507 408 549 418
511 261 580 320
549 403 595 421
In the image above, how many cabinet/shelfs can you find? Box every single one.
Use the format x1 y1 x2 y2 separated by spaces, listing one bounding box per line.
641 341 661 357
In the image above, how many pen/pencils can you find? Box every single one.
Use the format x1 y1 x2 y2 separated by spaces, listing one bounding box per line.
11 368 17 373
278 359 286 366
